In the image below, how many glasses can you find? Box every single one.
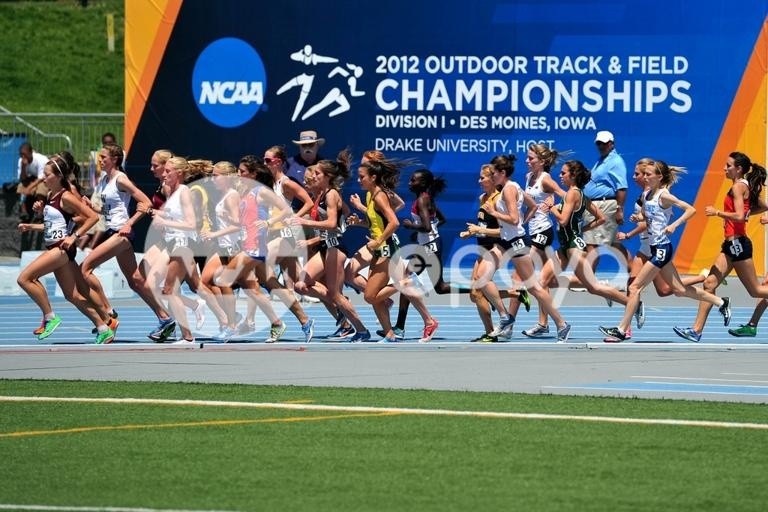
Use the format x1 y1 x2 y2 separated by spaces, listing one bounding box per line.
264 156 279 164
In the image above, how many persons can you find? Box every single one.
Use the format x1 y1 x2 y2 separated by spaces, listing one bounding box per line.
273 44 339 122
302 61 367 119
13 128 766 341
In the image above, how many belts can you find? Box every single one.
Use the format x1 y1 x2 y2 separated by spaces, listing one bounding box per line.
590 197 616 202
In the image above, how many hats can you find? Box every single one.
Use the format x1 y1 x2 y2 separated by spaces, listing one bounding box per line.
293 131 325 149
595 131 614 145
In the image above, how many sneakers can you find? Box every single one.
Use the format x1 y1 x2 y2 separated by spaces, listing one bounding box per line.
728 323 757 337
33 308 119 346
265 295 370 344
376 298 438 344
212 309 258 344
718 296 732 326
522 322 550 338
515 286 531 311
471 314 515 342
556 321 571 344
700 267 728 286
148 299 207 348
673 325 702 342
600 279 613 308
604 327 632 342
635 301 645 329
599 326 626 340
